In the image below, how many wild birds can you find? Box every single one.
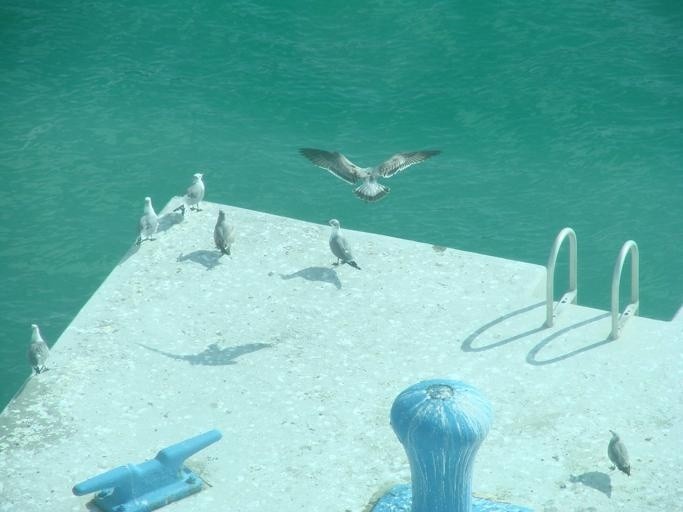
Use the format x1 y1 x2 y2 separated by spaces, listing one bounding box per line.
27 324 50 375
298 147 443 202
607 429 631 477
327 218 363 271
213 207 236 256
182 173 205 211
138 197 160 242
